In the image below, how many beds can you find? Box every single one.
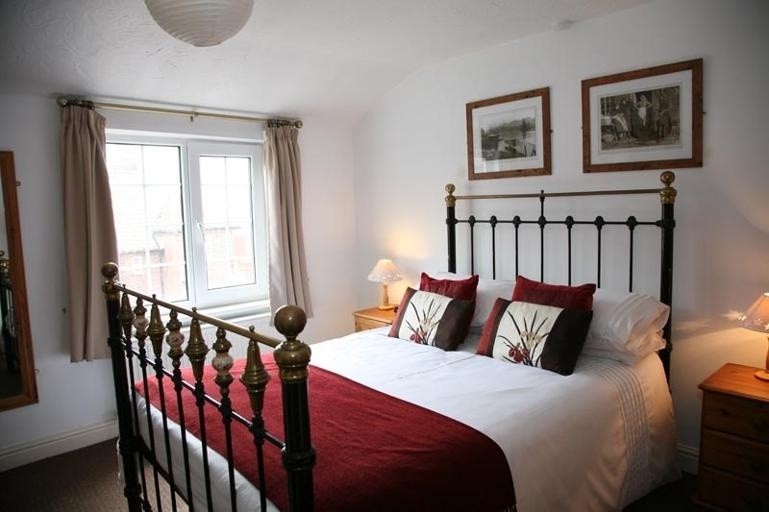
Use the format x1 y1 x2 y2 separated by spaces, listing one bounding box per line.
101 169 677 512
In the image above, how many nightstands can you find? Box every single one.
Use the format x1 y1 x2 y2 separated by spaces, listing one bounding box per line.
690 362 769 512
352 304 399 333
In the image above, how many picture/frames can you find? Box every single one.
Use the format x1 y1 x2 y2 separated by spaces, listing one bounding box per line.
466 86 552 181
582 58 704 175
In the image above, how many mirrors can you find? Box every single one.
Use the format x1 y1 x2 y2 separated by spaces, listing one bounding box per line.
0 151 39 411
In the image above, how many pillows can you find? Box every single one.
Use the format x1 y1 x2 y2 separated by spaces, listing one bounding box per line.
414 272 672 365
474 297 594 375
512 275 596 311
388 287 471 352
419 271 479 344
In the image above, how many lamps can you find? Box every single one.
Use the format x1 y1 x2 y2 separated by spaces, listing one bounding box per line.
736 293 769 382
367 259 403 310
